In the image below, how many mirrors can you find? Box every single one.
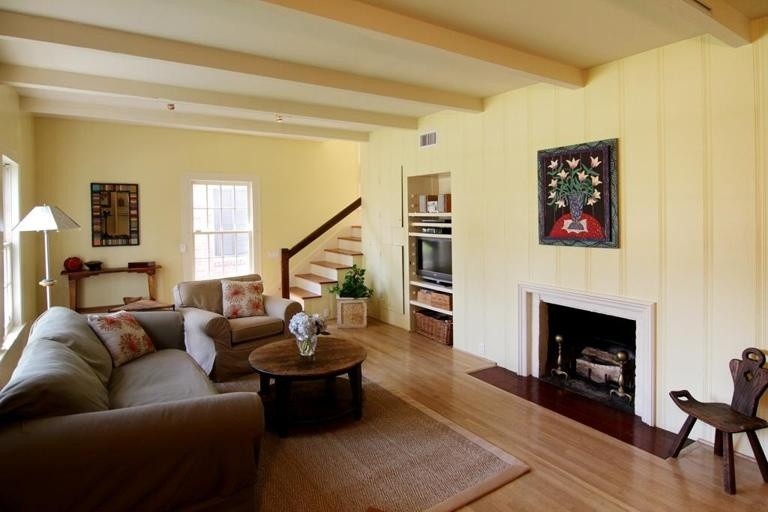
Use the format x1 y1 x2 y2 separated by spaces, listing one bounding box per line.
90 182 141 247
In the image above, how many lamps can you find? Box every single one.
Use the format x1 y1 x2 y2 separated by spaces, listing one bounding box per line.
12 202 81 311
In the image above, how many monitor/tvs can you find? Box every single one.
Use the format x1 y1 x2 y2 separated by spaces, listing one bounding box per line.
416 236 452 287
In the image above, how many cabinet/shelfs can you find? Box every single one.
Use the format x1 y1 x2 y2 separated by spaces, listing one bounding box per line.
408 212 453 318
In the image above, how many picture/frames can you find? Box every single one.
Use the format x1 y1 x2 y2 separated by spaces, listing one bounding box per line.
101 191 111 207
537 139 621 250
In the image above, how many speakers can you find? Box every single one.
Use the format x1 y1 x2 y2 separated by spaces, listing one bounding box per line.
438 194 451 212
418 194 427 213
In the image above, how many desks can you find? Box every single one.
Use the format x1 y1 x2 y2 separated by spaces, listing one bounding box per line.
59 264 162 314
549 213 603 239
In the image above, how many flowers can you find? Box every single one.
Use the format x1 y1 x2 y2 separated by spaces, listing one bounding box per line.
546 155 603 209
287 312 328 353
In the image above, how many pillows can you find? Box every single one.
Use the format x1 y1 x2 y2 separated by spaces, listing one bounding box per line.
220 279 264 319
85 310 157 369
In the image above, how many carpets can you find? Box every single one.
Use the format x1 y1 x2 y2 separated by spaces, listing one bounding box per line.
212 368 531 511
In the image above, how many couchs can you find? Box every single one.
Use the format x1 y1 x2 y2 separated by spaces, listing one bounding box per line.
0 305 267 511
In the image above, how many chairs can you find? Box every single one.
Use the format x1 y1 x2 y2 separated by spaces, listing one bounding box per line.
666 347 768 495
172 273 303 381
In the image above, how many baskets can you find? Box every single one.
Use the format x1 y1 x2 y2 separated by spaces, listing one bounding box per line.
412 307 453 345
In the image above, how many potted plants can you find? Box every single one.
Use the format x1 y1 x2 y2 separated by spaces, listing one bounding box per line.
326 264 375 328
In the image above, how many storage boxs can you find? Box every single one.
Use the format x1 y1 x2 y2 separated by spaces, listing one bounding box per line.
417 290 431 305
431 292 451 310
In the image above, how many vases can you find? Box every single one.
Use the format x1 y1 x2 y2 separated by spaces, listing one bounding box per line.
295 335 317 357
566 194 584 230
